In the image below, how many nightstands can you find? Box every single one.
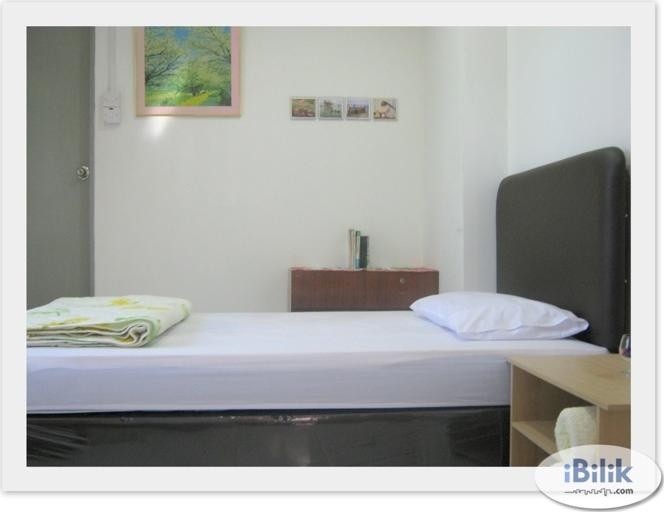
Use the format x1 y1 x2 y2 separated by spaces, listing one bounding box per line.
505 354 630 466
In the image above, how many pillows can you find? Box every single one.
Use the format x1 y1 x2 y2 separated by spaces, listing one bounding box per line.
406 287 590 344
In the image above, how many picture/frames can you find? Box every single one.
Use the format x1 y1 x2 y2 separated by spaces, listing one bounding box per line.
134 26 240 117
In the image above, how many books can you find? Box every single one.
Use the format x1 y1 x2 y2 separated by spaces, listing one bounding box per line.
355 230 361 269
347 229 355 271
359 236 368 268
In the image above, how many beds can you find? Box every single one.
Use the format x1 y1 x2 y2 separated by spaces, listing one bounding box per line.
27 147 630 466
